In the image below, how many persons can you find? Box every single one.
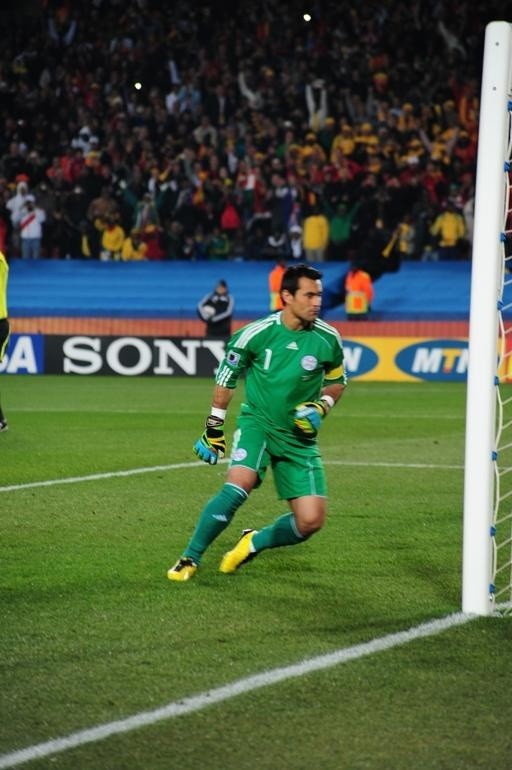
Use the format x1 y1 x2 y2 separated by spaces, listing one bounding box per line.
1 248 13 434
268 253 290 319
1 0 511 271
343 251 376 322
192 276 236 339
166 259 349 582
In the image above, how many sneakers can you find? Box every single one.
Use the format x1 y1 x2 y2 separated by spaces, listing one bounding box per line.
218 527 259 574
166 555 200 582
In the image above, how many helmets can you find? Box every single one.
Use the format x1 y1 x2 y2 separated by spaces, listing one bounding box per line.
191 415 227 466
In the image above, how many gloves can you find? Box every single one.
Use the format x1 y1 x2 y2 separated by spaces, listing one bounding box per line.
287 397 332 446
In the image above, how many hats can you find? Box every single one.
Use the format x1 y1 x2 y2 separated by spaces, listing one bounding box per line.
15 72 472 239
